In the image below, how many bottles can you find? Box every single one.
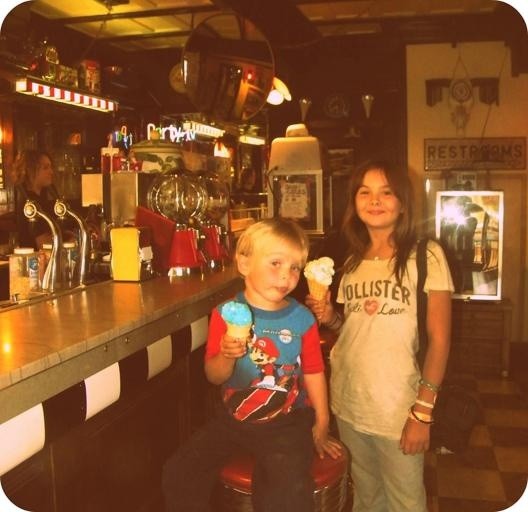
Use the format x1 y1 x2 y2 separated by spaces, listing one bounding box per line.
8 242 80 300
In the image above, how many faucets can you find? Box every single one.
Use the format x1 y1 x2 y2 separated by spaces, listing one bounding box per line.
24 198 61 291
55 197 90 289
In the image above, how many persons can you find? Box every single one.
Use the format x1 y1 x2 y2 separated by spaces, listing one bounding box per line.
9 147 76 252
161 219 343 512
306 161 455 512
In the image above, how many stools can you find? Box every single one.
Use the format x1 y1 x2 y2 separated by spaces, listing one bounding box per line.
223 429 352 511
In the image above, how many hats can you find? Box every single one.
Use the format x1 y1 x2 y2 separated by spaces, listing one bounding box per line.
253 335 281 357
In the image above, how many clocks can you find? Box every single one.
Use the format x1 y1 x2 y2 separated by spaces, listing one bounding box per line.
170 64 187 95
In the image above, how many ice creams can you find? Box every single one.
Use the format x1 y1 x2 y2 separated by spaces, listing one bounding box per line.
222 301 252 348
304 257 336 326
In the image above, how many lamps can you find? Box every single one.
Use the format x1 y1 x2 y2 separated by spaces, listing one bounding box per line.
267 74 294 105
14 76 121 114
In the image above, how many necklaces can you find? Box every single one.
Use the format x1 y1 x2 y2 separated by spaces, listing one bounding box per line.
373 254 381 259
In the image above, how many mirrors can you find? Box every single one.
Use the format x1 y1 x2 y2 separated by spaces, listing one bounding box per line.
179 14 275 122
437 190 505 301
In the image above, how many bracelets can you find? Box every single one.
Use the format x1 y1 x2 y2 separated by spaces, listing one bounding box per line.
409 378 440 422
324 312 343 331
